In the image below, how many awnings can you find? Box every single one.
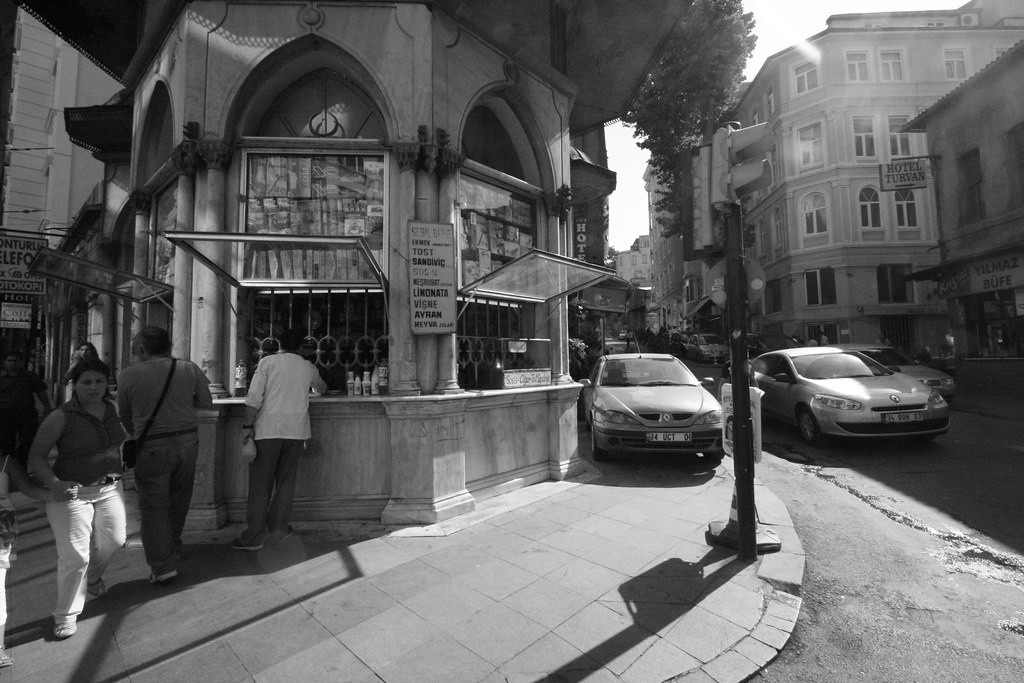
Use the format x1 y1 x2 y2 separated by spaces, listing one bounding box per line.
902 258 964 283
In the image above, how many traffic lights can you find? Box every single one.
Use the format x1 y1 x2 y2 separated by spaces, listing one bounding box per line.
709 122 775 213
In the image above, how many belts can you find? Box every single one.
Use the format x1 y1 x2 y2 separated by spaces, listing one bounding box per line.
97 476 121 485
143 427 198 440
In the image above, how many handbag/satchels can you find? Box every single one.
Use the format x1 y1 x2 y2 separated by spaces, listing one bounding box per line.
122 440 139 468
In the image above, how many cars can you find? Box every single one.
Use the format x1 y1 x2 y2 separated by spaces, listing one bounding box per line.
843 344 956 400
619 330 628 340
748 346 951 448
686 333 730 363
668 331 692 354
577 352 727 463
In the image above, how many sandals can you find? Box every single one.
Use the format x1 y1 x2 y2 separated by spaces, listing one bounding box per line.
0 646 13 667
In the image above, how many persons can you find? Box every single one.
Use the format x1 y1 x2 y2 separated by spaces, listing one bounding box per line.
117 326 213 584
819 330 829 346
625 329 632 347
0 452 79 668
27 357 129 639
61 342 115 401
875 337 893 347
0 349 51 467
791 333 804 345
633 324 671 353
807 335 818 347
915 345 932 363
231 330 327 552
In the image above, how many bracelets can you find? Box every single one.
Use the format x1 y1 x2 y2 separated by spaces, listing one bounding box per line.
243 424 255 429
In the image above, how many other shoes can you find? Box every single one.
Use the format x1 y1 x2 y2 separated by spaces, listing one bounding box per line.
150 561 176 583
279 525 293 543
88 578 106 596
170 538 183 560
54 622 77 638
232 537 262 550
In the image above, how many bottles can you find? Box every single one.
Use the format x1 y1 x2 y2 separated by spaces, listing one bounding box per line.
362 372 371 395
347 371 354 396
354 375 361 395
235 359 247 388
371 363 379 394
379 360 388 386
